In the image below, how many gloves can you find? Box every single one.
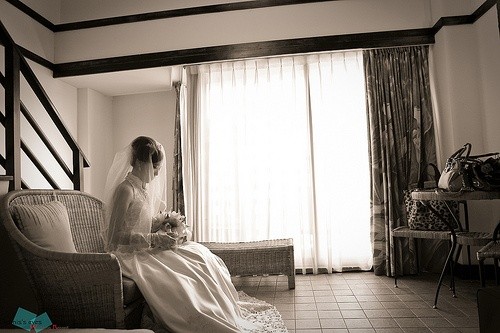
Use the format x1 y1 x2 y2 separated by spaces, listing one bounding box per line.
129 230 178 250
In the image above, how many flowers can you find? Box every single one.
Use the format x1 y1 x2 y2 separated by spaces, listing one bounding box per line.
152 211 185 246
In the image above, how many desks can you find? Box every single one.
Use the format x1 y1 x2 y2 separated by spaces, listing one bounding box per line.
409 190 499 309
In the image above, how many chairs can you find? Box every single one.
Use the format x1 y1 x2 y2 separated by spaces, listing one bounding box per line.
392 200 469 287
476 223 499 288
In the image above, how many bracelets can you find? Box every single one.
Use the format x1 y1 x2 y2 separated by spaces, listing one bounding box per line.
150 234 157 249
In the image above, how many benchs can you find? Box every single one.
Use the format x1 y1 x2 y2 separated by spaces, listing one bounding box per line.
2 189 134 328
196 238 295 289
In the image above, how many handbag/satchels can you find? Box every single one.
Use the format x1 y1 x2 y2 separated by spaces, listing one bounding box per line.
437 143 500 192
403 163 459 230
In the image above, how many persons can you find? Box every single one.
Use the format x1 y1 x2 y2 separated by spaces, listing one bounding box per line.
101 136 289 333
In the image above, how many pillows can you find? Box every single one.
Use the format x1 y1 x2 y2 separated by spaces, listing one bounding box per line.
13 200 77 254
